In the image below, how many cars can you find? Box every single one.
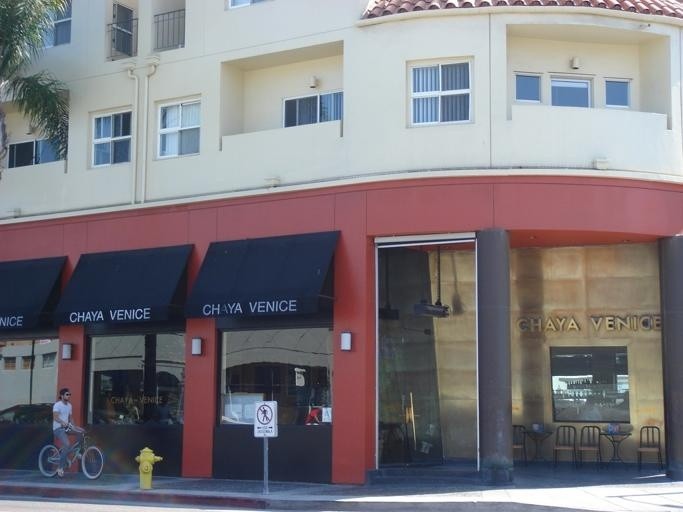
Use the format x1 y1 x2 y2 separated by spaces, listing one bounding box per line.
0 404 133 425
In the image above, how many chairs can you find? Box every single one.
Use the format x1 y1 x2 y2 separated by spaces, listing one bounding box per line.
512 424 527 466
552 425 602 472
635 426 662 473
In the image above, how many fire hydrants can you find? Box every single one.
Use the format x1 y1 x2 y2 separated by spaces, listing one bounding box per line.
134 447 164 491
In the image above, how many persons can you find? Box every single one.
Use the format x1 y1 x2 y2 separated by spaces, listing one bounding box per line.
50 386 88 479
158 395 178 424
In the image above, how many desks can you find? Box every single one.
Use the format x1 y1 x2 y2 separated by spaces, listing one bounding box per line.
522 431 553 467
599 431 632 472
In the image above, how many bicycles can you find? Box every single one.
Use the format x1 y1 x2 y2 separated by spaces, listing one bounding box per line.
36 422 105 481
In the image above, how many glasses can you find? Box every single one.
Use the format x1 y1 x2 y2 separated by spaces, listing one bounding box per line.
66 393 72 397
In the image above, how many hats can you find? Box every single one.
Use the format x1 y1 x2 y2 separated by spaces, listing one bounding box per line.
58 388 67 399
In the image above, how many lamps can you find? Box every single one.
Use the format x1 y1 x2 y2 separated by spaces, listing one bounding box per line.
191 336 202 355
339 331 351 351
61 343 72 360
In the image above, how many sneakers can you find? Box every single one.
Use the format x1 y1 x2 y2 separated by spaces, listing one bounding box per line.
56 451 82 478
